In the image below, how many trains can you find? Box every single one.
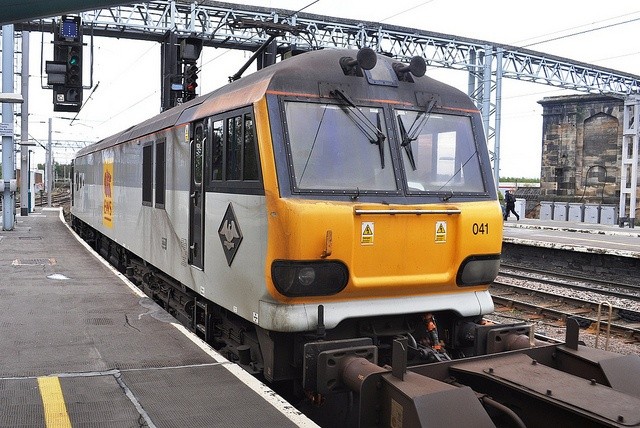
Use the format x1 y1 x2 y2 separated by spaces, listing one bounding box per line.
68 18 504 392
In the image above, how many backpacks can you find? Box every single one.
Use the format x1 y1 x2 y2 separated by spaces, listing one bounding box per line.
507 194 516 201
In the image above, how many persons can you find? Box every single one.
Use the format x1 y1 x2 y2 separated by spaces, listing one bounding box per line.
504 190 519 221
